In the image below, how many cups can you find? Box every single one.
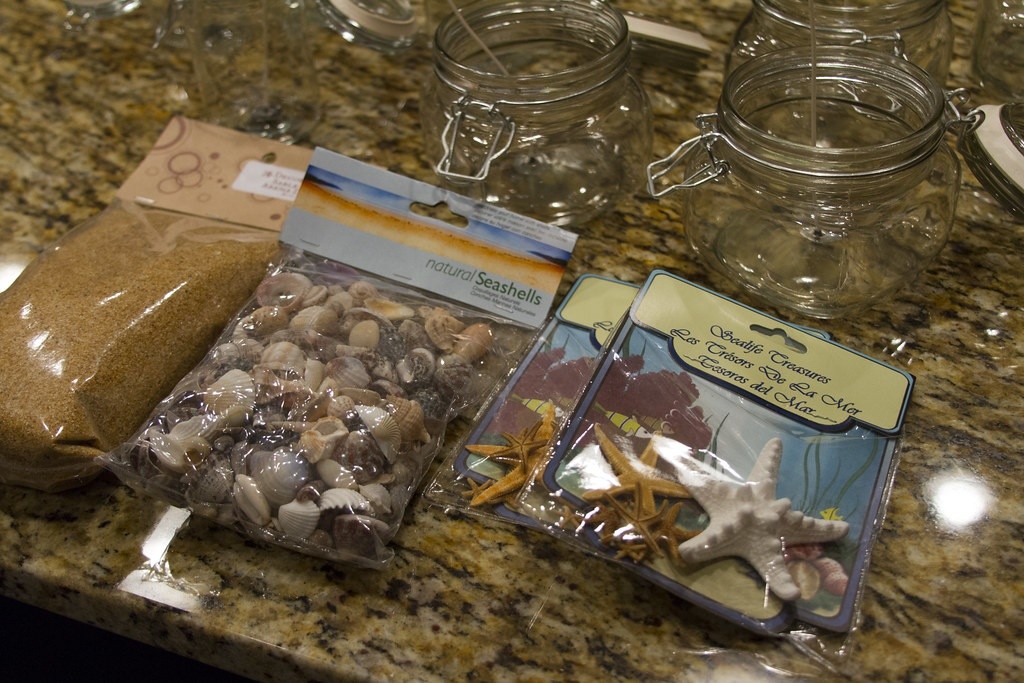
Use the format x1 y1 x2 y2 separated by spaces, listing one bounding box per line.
168 0 1024 318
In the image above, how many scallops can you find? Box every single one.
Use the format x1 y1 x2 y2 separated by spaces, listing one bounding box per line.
131 273 494 557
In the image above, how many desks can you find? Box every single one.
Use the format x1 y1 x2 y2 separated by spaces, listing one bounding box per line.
0 1 1021 679
